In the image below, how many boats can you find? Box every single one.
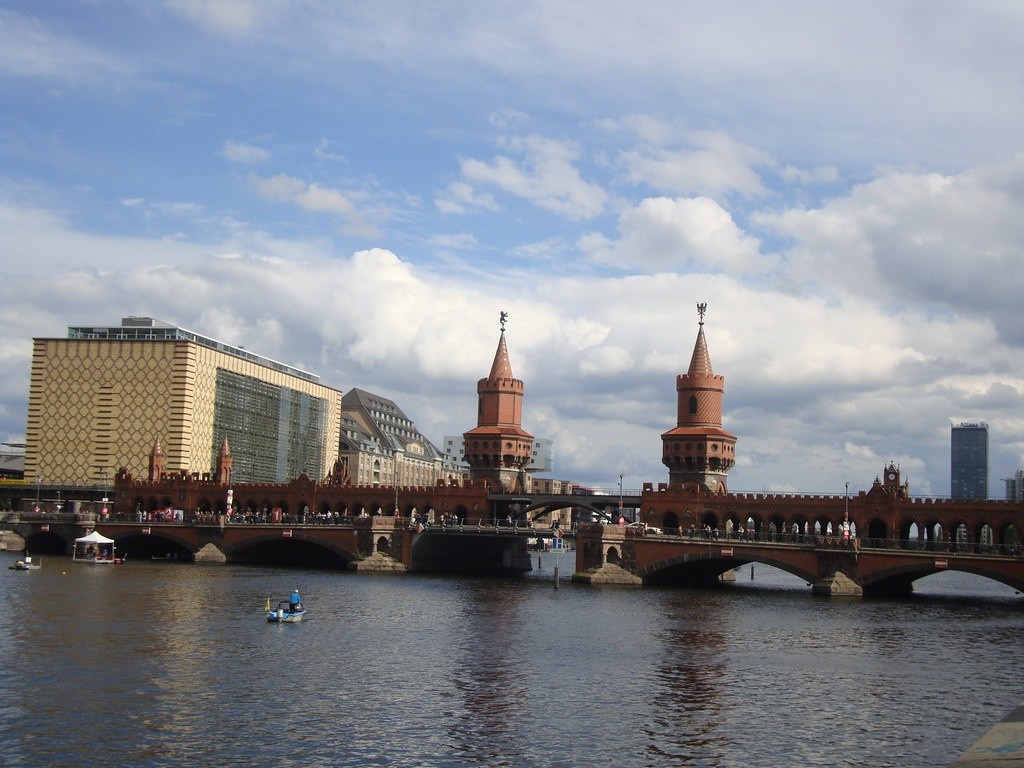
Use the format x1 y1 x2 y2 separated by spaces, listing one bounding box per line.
152 553 187 561
72 530 128 564
9 557 43 571
266 601 306 623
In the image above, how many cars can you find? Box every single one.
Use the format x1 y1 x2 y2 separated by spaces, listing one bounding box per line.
627 521 664 536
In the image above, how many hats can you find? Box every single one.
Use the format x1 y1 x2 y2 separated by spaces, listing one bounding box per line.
294 590 298 592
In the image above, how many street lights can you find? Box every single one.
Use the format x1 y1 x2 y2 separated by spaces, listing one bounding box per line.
103 472 110 516
617 472 625 525
844 481 851 538
394 472 400 518
36 474 41 514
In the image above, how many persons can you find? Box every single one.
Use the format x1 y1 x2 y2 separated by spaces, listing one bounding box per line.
527 518 533 529
193 508 357 527
506 514 514 528
138 509 179 520
102 549 107 556
676 523 682 538
549 519 560 528
289 589 300 611
410 512 460 529
701 523 719 541
738 524 754 543
687 523 696 537
